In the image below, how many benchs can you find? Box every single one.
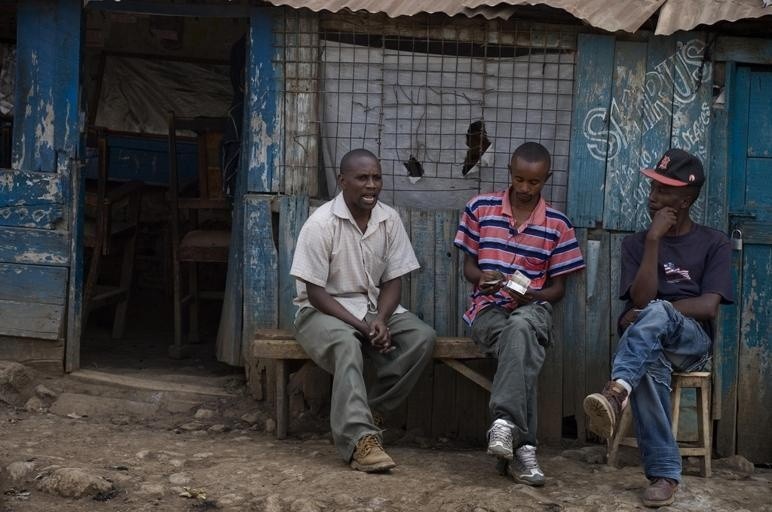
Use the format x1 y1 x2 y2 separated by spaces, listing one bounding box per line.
251 329 501 446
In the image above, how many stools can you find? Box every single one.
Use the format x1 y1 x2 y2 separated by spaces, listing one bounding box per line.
602 368 716 480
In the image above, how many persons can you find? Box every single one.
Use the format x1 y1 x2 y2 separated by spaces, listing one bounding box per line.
451 140 587 489
579 147 736 509
287 148 439 476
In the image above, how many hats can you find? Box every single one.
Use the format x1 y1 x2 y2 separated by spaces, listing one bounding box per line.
639 147 706 188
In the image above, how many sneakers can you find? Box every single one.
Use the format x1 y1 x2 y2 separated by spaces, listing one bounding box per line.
484 419 516 461
371 410 398 449
582 381 629 441
642 477 680 508
498 444 547 488
348 432 400 473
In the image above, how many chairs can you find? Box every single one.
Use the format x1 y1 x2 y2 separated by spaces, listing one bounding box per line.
164 108 237 359
80 138 145 340
